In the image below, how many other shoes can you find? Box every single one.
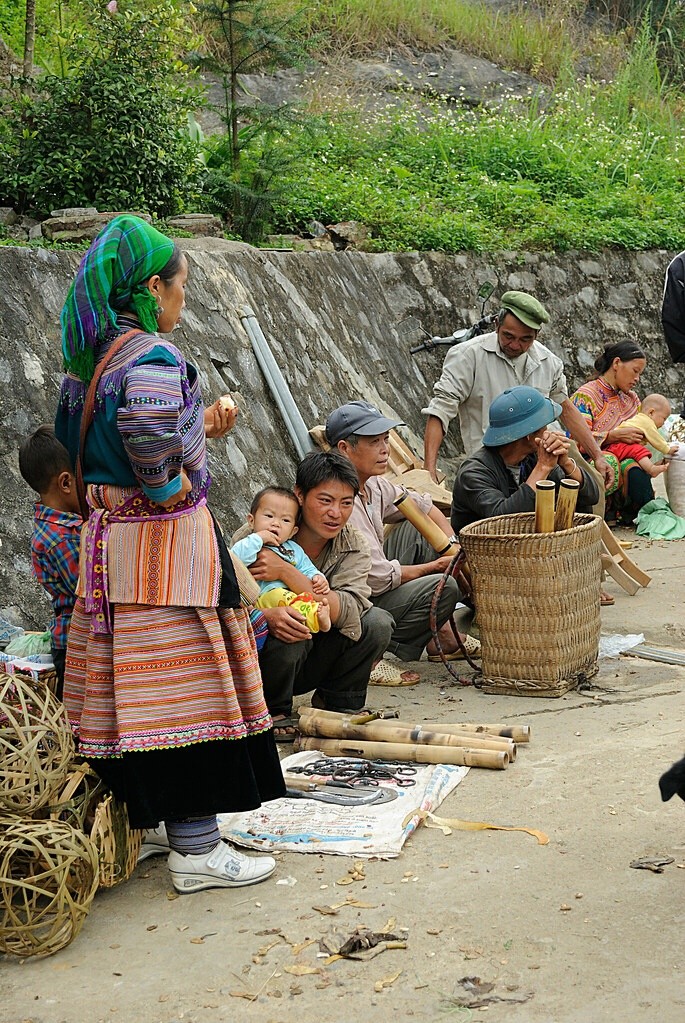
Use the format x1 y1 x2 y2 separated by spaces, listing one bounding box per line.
605 514 617 526
601 587 615 604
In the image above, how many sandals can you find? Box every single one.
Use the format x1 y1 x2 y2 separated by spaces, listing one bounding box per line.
272 717 300 741
342 707 372 716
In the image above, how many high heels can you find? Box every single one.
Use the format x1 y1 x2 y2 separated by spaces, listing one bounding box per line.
168 840 276 895
136 821 172 863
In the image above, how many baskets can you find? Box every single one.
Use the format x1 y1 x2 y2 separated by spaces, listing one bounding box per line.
459 512 602 698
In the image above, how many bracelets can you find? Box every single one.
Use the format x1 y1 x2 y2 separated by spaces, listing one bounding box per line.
568 457 577 476
447 535 461 544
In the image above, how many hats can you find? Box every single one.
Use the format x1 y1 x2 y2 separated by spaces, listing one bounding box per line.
326 401 407 447
482 386 562 446
498 290 550 329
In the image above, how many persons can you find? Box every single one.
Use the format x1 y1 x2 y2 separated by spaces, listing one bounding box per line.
590 393 679 479
18 423 83 694
568 339 656 523
421 291 615 606
661 251 685 365
451 386 615 606
324 401 481 687
232 485 333 633
60 214 288 894
229 450 397 742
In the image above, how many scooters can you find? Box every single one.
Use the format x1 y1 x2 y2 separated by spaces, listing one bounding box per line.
398 280 503 356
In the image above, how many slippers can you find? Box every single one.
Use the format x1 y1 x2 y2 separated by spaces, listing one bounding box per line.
427 634 481 661
368 658 420 687
